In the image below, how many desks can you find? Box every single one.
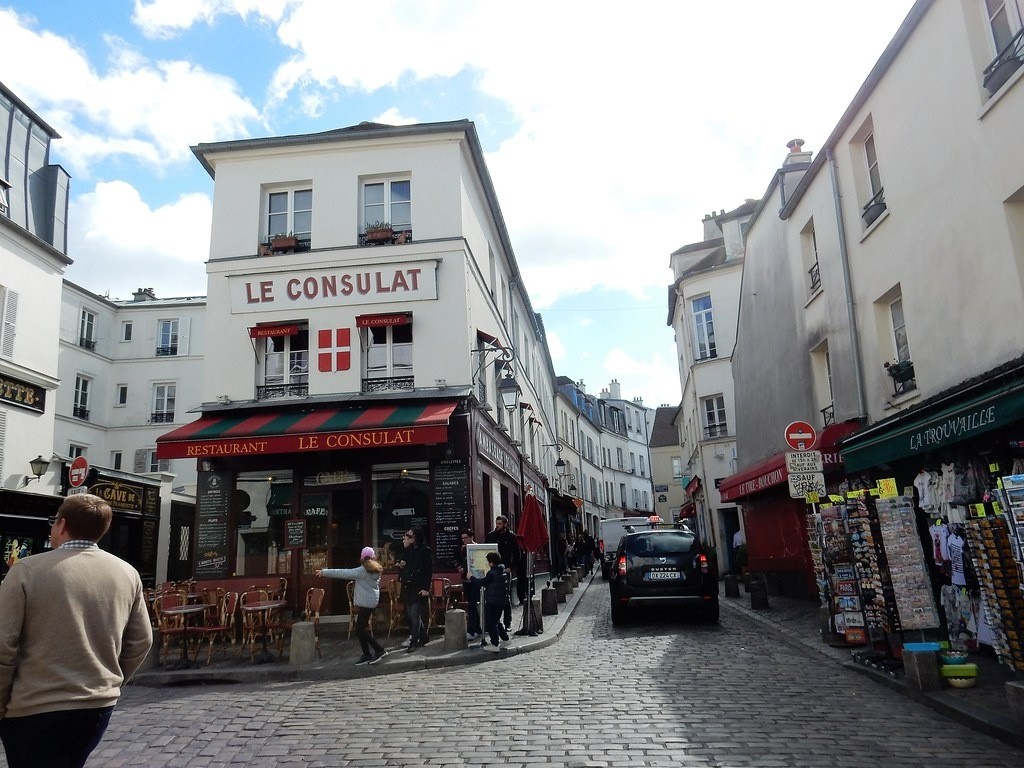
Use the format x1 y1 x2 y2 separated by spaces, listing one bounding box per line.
380 583 464 603
240 600 287 665
159 605 209 671
148 595 199 602
238 591 272 595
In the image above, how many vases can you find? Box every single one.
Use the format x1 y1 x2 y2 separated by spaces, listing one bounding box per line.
861 203 886 226
893 366 915 383
982 58 1022 95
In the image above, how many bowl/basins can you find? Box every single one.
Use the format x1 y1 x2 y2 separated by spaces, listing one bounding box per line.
940 654 969 664
947 678 976 687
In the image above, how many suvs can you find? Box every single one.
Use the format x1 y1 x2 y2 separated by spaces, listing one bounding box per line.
604 516 720 626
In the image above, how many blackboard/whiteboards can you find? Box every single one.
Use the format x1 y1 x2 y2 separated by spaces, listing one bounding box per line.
285 519 307 549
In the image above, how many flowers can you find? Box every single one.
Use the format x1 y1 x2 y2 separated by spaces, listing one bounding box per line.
884 358 913 377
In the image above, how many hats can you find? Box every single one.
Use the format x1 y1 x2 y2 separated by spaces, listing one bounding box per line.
361 547 374 558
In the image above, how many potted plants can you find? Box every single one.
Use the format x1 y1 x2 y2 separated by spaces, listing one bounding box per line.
271 231 298 249
365 218 393 241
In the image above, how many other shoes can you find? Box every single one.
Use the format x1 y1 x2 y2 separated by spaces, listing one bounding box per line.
406 641 419 653
417 639 430 647
473 632 482 639
467 633 474 640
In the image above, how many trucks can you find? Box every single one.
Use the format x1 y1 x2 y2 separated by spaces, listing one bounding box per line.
599 516 659 582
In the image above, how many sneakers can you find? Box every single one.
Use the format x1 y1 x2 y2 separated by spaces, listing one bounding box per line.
354 654 373 665
369 648 389 664
483 643 500 652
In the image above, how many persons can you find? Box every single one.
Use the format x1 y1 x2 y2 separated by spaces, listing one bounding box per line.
556 529 599 575
733 530 742 551
316 547 388 665
456 528 482 640
395 525 433 652
486 515 528 605
0 493 154 768
466 552 512 652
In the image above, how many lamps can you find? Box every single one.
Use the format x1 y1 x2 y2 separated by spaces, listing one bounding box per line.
25 455 50 486
215 395 231 404
471 347 523 417
494 423 508 431
508 440 523 446
434 379 447 389
559 474 576 490
542 444 566 466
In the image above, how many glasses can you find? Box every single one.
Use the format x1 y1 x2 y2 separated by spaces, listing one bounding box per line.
462 537 471 540
49 516 64 526
406 534 415 538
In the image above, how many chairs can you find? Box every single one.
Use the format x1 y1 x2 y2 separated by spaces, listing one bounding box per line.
387 579 410 639
143 582 325 666
346 581 379 640
427 578 458 636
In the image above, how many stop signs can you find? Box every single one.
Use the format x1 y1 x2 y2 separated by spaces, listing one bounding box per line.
68 456 88 488
784 421 817 451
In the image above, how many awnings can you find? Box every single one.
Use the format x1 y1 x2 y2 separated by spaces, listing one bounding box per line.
718 423 859 503
679 503 697 518
838 378 1024 477
155 402 459 460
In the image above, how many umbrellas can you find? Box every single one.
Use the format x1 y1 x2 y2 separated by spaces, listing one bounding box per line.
515 491 549 629
